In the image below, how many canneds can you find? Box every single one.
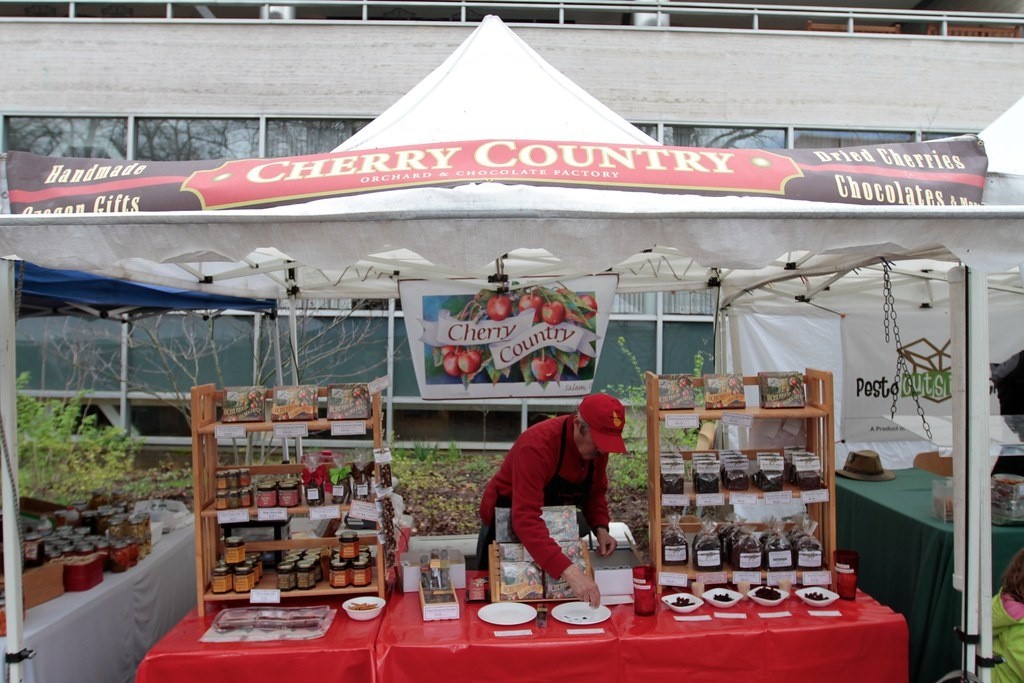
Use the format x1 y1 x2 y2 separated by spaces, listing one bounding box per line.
24 488 151 591
215 467 301 510
211 536 263 594
327 531 372 589
277 547 323 592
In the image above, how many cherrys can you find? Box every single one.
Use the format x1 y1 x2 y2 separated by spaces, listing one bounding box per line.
438 293 596 381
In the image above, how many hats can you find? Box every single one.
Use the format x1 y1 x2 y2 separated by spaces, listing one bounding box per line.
578 393 627 454
835 450 896 481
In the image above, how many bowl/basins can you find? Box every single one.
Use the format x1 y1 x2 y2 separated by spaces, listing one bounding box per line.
794 586 841 607
660 592 705 614
341 596 386 621
746 586 789 607
701 587 744 609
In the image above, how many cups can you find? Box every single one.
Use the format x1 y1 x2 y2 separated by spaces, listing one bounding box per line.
779 580 792 600
633 566 657 615
737 582 750 601
832 549 859 600
691 582 704 599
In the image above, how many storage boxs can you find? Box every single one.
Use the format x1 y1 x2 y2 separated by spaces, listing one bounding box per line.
150 522 164 543
932 479 953 523
396 549 466 592
0 561 64 609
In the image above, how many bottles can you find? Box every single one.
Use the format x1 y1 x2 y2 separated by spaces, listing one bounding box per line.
54 489 152 573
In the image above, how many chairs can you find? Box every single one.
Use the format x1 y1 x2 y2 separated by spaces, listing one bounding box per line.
913 452 953 479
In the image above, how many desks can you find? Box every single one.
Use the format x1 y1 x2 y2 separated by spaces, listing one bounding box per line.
0 518 196 683
835 467 1024 683
134 571 909 683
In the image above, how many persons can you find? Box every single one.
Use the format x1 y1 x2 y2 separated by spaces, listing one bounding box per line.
991 548 1024 683
476 394 628 609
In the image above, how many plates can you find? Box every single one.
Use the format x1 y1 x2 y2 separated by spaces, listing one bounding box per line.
477 602 537 626
550 601 612 626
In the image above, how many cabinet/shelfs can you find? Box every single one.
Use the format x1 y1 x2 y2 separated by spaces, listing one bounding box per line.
190 377 391 616
646 369 838 592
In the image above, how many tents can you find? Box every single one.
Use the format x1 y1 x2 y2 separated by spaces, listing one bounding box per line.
0 14 1024 683
